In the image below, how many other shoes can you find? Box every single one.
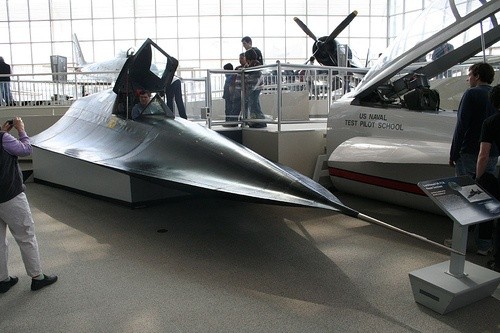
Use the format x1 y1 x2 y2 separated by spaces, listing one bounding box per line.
31 273 58 291
0 276 19 293
476 245 494 256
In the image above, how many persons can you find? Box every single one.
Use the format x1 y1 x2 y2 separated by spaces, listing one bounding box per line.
431 42 500 255
0 56 16 106
304 57 321 100
0 117 57 294
151 65 188 119
131 90 164 120
222 37 267 128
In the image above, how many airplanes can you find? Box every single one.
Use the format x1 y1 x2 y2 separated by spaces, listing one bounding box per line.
292 9 372 82
44 32 194 84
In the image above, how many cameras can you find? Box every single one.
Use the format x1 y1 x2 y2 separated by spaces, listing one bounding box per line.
7 119 17 126
